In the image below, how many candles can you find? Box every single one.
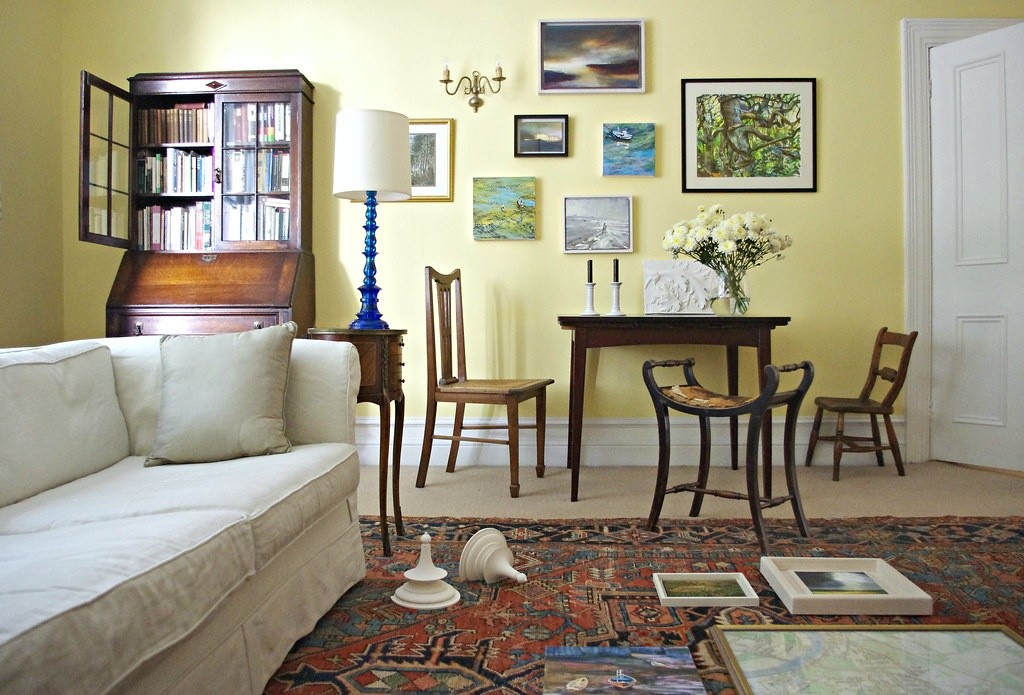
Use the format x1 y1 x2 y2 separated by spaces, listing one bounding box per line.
613 258 619 282
587 259 593 283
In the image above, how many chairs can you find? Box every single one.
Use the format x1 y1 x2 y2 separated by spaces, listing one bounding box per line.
416 266 556 499
805 326 919 481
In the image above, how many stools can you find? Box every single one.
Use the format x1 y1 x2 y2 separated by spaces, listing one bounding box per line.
643 357 816 556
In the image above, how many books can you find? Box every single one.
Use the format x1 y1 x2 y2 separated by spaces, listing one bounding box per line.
132 147 216 192
134 199 216 250
224 103 292 142
134 102 216 143
221 195 290 241
223 149 291 193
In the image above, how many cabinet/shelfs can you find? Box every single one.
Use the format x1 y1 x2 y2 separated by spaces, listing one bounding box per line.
77 69 315 337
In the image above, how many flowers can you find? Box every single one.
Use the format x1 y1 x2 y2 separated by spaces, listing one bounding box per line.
661 204 794 314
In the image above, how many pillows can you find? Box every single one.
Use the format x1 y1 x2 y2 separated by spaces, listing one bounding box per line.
145 322 298 465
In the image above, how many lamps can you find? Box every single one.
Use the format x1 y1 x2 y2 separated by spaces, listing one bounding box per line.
441 54 506 115
333 110 410 328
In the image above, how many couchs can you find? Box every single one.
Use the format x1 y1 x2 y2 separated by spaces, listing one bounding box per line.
1 329 366 694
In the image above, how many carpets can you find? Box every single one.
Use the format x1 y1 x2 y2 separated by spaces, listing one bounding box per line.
261 514 1024 694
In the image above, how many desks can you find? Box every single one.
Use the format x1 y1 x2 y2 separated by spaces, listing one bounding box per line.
555 312 790 506
307 329 407 557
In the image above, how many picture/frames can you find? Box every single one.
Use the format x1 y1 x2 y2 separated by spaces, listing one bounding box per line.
537 19 647 94
561 195 633 254
512 114 569 157
758 555 933 616
680 79 818 192
351 118 455 202
653 572 760 608
708 624 1023 694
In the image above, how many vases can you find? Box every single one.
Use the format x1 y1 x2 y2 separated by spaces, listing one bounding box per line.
725 274 748 315
